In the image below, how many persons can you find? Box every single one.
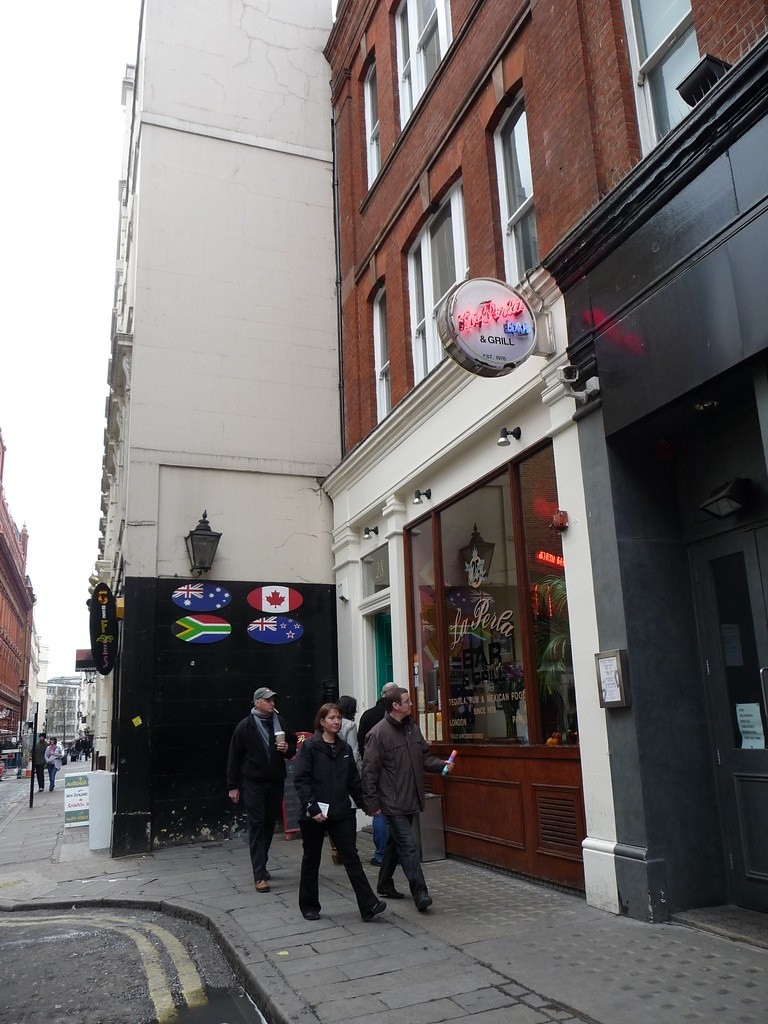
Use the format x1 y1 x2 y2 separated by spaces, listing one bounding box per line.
29 733 62 792
75 736 93 762
4 738 27 769
226 688 298 893
361 688 455 913
329 682 412 869
293 703 387 922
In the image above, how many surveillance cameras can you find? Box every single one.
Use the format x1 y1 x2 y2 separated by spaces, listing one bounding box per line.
557 365 579 383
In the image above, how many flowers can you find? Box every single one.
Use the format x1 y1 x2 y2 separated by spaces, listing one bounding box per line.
486 661 525 737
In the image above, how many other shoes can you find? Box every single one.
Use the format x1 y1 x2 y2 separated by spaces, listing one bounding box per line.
39 788 43 791
370 858 382 866
361 900 386 921
417 896 432 912
50 785 54 791
377 889 404 898
299 905 320 920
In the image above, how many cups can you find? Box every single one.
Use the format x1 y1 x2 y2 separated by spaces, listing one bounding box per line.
274 730 285 746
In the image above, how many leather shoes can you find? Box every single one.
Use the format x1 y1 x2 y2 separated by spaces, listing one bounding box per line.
255 880 270 892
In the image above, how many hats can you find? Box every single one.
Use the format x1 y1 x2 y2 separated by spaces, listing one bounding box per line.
254 688 277 700
381 682 398 698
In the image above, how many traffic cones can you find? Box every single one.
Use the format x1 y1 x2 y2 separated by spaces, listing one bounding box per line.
24 758 32 777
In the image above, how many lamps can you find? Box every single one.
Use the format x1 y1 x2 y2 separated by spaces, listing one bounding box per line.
413 488 431 504
498 426 522 447
184 509 222 577
461 523 495 584
674 53 734 108
362 526 378 540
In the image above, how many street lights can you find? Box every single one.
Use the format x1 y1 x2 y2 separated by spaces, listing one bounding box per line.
44 709 49 736
18 680 26 778
